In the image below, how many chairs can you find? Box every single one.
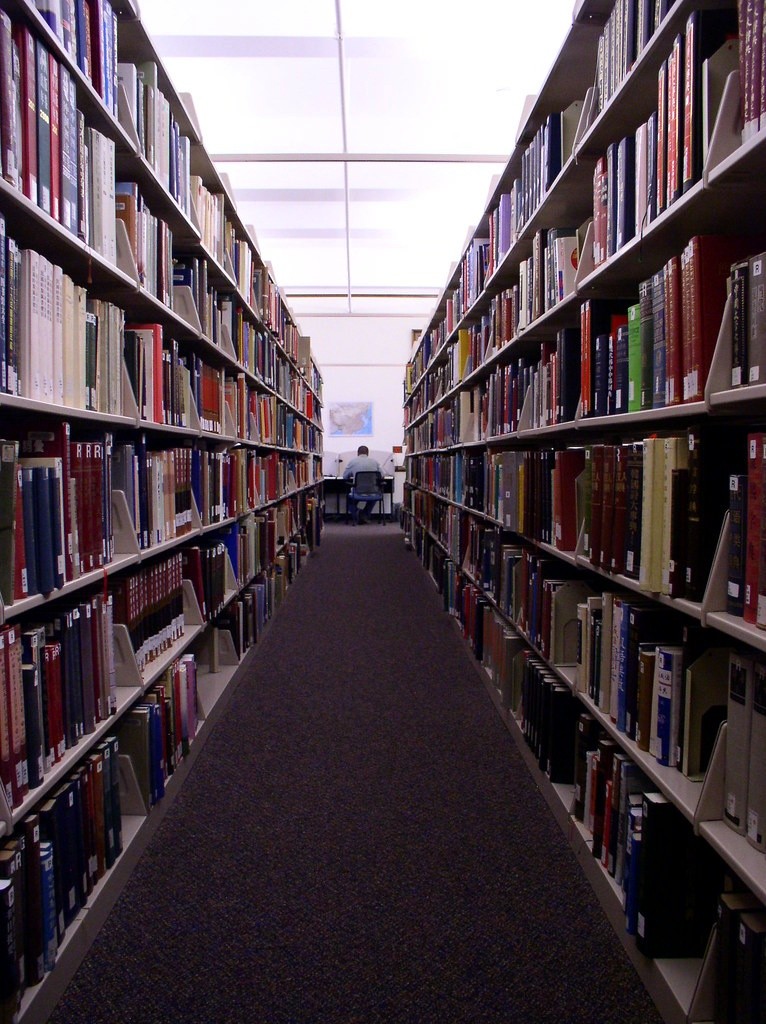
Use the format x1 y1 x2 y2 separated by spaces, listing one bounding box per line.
346 471 386 527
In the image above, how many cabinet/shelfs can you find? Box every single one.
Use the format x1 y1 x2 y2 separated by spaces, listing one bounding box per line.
404 0 766 1024
1 1 322 1024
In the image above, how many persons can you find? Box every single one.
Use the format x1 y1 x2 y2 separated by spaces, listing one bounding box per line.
343 446 383 525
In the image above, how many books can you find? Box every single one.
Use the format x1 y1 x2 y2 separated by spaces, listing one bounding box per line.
31 0 323 403
399 0 766 1024
0 9 321 429
0 214 324 1024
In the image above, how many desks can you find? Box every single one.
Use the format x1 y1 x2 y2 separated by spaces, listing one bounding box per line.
324 477 395 523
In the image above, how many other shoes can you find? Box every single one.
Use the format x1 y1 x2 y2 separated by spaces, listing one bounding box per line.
356 510 371 524
